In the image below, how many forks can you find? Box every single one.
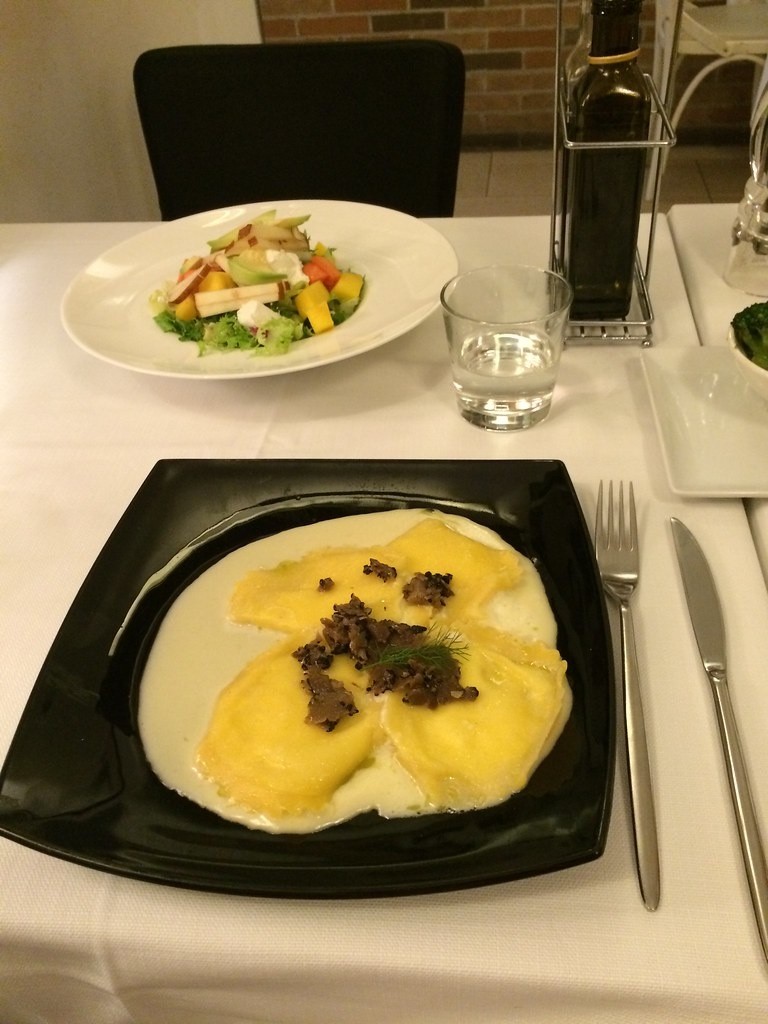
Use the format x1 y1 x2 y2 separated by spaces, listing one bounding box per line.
592 480 660 912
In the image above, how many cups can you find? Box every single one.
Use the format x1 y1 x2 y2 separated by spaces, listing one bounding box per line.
441 262 573 431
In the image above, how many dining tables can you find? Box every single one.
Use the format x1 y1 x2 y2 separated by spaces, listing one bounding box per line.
0 206 768 1024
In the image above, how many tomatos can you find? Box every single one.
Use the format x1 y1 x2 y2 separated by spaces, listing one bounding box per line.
303 256 342 290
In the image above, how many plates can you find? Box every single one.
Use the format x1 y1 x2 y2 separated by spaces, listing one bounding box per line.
2 459 617 900
638 347 768 498
58 199 458 382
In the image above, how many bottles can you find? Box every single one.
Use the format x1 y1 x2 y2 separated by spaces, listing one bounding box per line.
556 0 651 321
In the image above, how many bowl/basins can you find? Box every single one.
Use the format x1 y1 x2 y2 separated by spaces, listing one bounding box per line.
729 325 768 403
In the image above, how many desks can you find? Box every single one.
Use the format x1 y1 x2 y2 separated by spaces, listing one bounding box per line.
642 0 768 198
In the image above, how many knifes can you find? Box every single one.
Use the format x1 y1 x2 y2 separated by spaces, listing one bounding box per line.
670 517 767 963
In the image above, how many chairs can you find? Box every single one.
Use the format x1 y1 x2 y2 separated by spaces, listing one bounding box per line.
134 38 467 224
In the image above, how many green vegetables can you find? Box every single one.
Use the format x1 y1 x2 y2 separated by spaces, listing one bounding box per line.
729 300 768 371
148 280 360 359
358 622 474 677
